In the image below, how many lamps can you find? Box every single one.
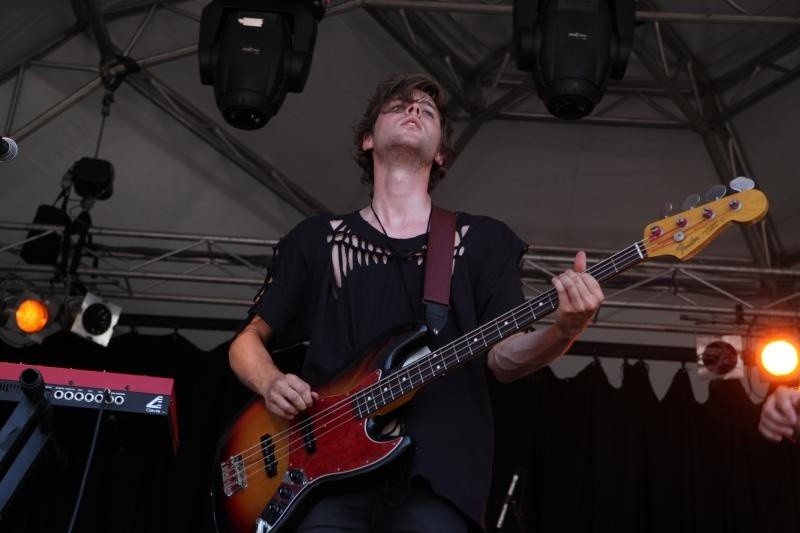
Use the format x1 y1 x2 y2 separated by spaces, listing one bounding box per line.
693 303 800 382
0 269 124 349
199 0 326 129
513 0 636 123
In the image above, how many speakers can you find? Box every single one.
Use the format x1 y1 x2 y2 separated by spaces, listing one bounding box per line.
74 157 115 201
23 204 71 266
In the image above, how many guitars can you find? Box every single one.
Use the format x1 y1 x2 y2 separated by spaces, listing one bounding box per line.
199 175 770 533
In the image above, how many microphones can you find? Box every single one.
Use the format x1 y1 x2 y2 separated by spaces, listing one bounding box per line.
0 137 18 162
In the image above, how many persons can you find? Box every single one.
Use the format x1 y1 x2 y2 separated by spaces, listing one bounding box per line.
759 385 800 442
229 74 605 533
